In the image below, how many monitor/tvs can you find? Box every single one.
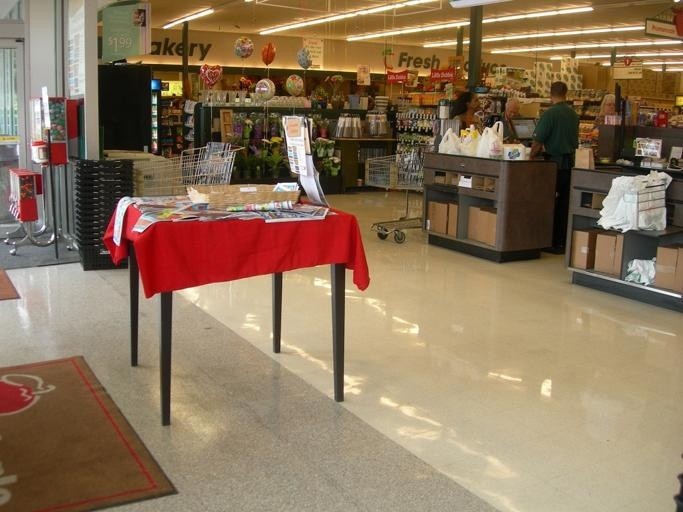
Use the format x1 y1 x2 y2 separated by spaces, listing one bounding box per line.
509 118 536 141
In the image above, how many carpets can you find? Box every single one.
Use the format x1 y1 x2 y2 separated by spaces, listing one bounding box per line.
1 265 21 300
0 357 179 512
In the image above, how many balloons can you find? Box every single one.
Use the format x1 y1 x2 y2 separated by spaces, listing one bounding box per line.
199 63 224 89
261 41 277 67
295 46 313 70
234 36 255 59
254 78 276 102
285 73 304 96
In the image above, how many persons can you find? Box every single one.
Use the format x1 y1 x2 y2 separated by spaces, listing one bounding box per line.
496 95 522 144
528 81 579 254
667 105 683 128
448 90 486 138
592 93 617 129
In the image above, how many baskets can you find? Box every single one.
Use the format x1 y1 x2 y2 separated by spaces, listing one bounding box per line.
186 182 301 212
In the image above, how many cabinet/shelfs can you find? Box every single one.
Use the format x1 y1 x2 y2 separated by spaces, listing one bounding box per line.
564 165 683 311
201 105 367 186
183 101 202 155
161 96 182 157
422 152 558 264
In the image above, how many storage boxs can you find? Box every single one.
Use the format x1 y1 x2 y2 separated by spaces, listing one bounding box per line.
593 234 623 276
572 228 596 270
448 203 457 238
480 208 497 243
520 102 540 118
655 244 683 293
427 201 448 236
468 206 489 242
488 212 497 248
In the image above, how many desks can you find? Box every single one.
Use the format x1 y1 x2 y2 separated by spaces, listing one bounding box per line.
331 138 398 193
121 196 357 427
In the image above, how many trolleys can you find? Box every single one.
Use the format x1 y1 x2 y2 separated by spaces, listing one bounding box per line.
365 142 425 243
135 143 246 194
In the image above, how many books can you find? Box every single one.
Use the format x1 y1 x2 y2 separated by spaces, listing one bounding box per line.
112 194 335 247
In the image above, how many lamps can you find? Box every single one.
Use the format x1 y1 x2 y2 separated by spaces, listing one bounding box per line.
346 6 594 41
491 41 682 55
449 0 507 9
162 7 217 30
550 53 683 61
424 26 636 48
602 61 683 66
258 0 442 35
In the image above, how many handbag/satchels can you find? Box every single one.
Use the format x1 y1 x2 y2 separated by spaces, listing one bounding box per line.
574 144 594 170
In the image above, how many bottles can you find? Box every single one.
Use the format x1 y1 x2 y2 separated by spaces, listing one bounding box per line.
653 108 671 129
